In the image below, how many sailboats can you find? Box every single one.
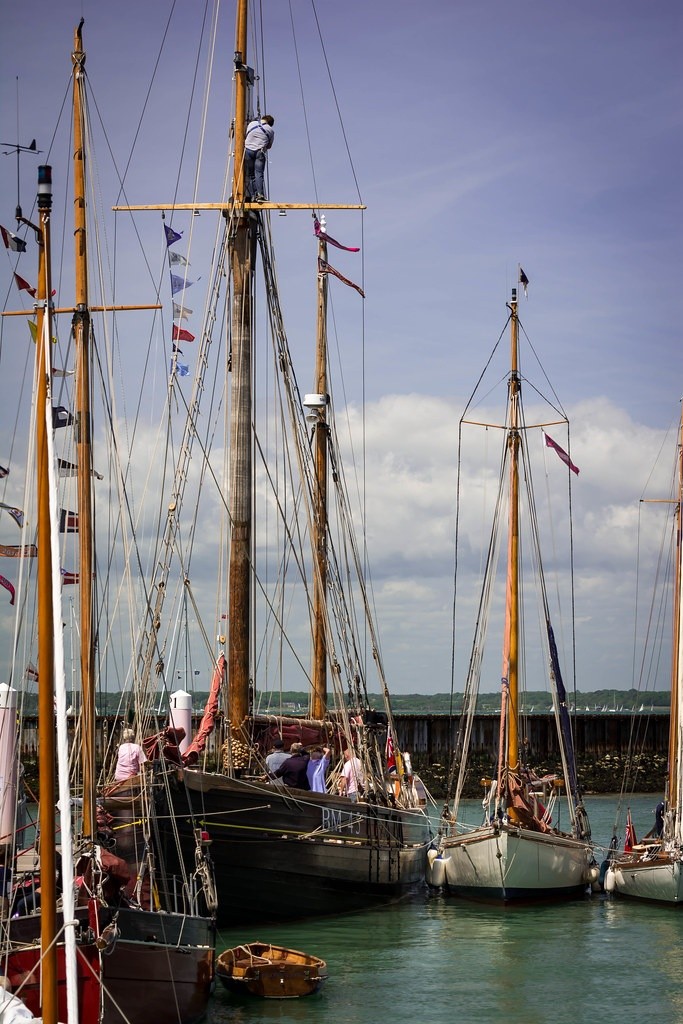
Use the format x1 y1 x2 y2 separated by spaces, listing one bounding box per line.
0 0 439 1024
436 267 595 907
607 396 683 904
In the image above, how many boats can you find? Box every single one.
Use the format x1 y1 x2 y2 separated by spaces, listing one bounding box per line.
214 941 329 998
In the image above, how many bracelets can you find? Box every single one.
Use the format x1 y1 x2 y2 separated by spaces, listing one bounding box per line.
322 747 324 750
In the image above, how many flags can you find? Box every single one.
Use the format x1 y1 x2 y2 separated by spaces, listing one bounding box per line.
314 219 360 253
51 406 78 429
0 543 38 558
163 226 195 376
0 224 27 253
28 319 57 344
624 815 637 851
0 501 29 528
545 434 580 476
59 508 78 533
15 274 56 299
385 737 396 773
0 575 15 605
58 459 104 480
25 661 39 682
318 257 365 300
61 568 79 585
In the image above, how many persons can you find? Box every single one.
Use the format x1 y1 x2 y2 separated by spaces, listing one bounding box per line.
267 743 311 791
656 801 664 839
115 729 147 781
266 740 292 786
306 746 332 794
339 749 365 798
244 115 275 202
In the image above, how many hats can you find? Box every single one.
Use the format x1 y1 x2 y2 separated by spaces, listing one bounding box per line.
273 740 284 748
